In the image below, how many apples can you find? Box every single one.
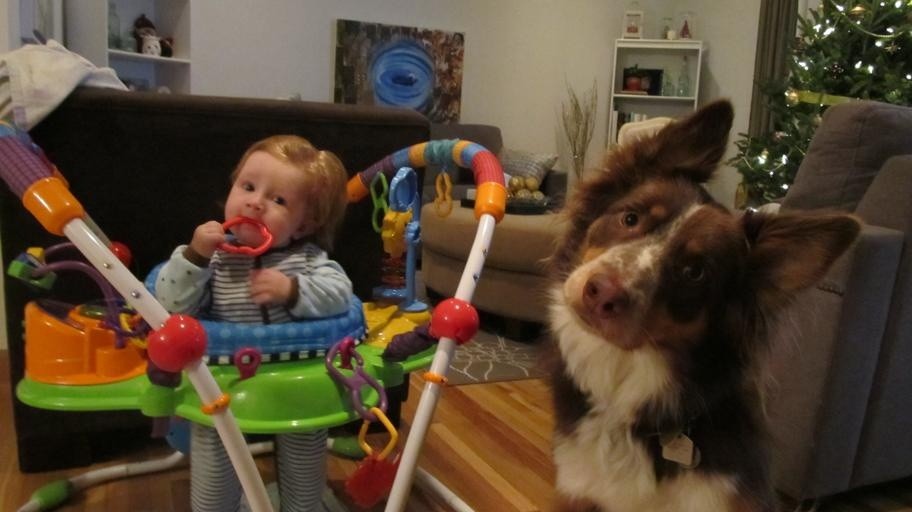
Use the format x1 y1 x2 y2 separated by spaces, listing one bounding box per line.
506 175 546 202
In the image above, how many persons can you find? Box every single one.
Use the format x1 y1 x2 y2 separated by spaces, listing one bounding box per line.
155 134 352 512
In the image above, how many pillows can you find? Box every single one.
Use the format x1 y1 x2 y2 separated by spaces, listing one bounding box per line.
496 147 559 183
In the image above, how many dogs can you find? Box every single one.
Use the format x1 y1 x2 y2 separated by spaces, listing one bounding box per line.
532 98 864 512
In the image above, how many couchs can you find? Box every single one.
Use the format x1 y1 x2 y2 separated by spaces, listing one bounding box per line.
418 125 573 324
9 100 426 470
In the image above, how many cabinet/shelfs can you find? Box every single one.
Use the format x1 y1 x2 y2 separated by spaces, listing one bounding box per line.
61 3 190 102
607 36 703 149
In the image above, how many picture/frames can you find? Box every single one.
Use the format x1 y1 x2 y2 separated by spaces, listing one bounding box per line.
623 11 643 38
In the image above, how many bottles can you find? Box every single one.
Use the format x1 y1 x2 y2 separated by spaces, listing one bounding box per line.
109 0 121 52
662 55 689 97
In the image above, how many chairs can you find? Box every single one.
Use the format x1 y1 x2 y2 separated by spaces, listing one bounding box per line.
738 105 910 510
